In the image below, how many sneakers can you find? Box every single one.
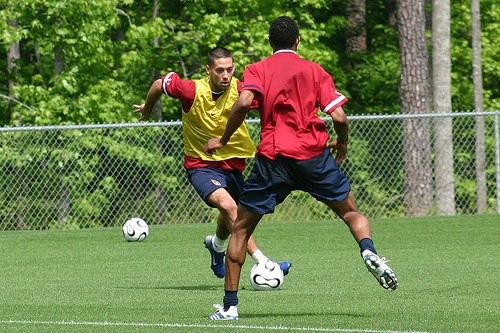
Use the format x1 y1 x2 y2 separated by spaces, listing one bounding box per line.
276 261 291 275
208 304 239 321
362 250 399 291
204 236 226 278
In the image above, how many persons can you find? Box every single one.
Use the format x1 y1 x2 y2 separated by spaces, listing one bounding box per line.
133 47 292 279
201 15 399 321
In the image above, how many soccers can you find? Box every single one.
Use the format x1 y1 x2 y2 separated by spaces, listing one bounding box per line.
250 259 284 291
122 218 149 241
